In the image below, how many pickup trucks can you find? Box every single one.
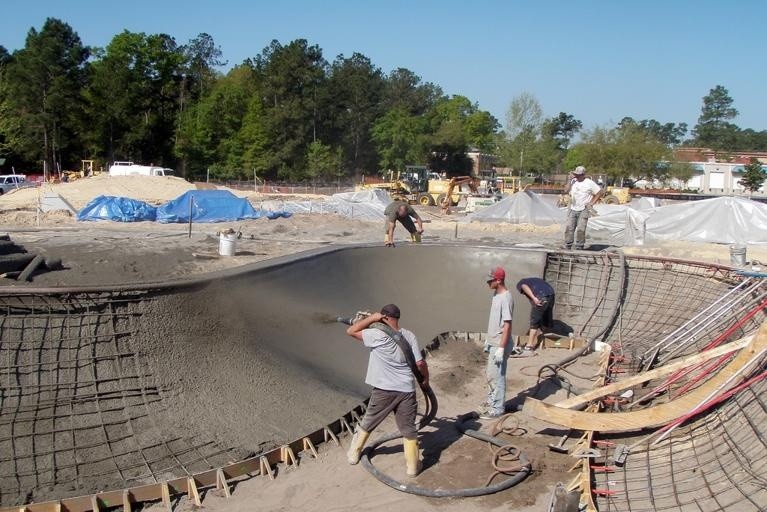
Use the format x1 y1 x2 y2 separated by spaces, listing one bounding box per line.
0 175 36 194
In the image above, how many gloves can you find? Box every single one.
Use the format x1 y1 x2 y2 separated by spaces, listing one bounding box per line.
493 348 505 364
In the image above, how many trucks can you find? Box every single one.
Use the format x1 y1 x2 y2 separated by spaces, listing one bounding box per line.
109 160 176 176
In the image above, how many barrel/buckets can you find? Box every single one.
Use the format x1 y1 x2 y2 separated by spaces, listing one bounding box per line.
218 229 240 256
729 244 748 270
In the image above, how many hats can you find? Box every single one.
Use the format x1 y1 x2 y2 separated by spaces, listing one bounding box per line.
381 304 400 319
573 166 585 175
487 268 505 282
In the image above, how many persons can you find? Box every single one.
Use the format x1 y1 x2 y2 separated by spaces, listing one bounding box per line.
384 201 424 246
347 303 430 477
517 277 555 351
61 173 70 183
479 268 514 420
86 163 90 178
564 166 602 249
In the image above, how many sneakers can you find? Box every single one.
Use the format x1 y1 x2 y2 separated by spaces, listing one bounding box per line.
521 343 535 351
565 242 572 250
479 410 501 420
479 402 492 408
575 244 584 250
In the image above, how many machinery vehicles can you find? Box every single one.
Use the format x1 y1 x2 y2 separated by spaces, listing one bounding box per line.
355 164 638 209
63 159 106 181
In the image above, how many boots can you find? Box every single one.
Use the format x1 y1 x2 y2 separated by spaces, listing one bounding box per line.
347 423 370 465
403 436 423 478
